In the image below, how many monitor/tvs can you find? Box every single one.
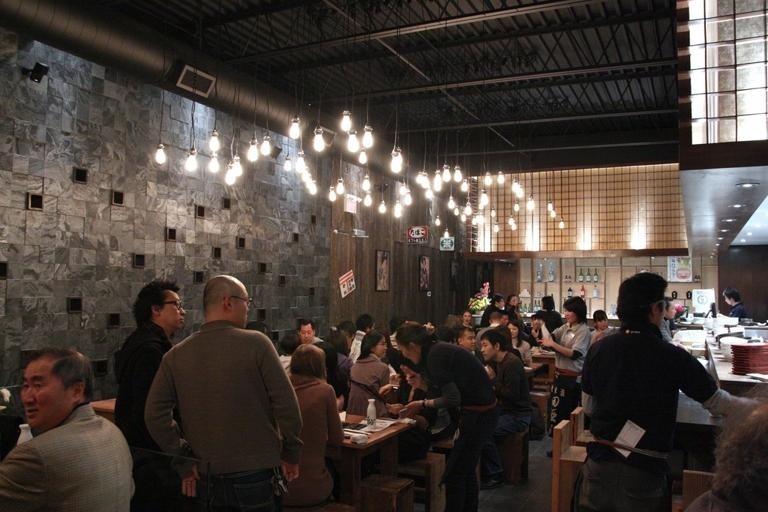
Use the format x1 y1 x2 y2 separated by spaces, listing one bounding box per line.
711 303 716 317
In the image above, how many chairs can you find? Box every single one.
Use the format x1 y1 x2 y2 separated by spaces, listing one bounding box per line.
551 406 595 512
672 468 716 511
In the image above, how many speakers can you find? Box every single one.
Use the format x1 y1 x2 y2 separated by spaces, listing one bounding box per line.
379 183 389 192
270 144 282 159
30 62 50 83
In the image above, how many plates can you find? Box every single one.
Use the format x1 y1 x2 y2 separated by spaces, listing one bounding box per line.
719 336 746 360
730 343 768 375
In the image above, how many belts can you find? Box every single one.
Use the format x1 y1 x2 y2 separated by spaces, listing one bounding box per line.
461 399 497 411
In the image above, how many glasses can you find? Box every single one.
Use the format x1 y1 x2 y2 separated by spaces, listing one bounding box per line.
232 295 252 303
164 301 183 309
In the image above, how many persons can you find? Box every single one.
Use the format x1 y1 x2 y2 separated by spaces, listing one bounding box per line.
112 276 187 511
143 275 305 511
244 293 620 511
420 257 428 290
575 273 752 512
657 296 677 343
721 285 745 320
683 397 767 512
376 251 389 291
0 347 136 511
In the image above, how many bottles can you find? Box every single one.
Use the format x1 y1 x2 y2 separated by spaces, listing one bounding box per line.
566 287 572 298
577 268 583 281
591 285 598 298
585 268 591 283
592 268 598 282
563 274 571 282
366 398 377 429
536 262 543 283
547 262 555 283
580 286 584 298
519 299 542 312
